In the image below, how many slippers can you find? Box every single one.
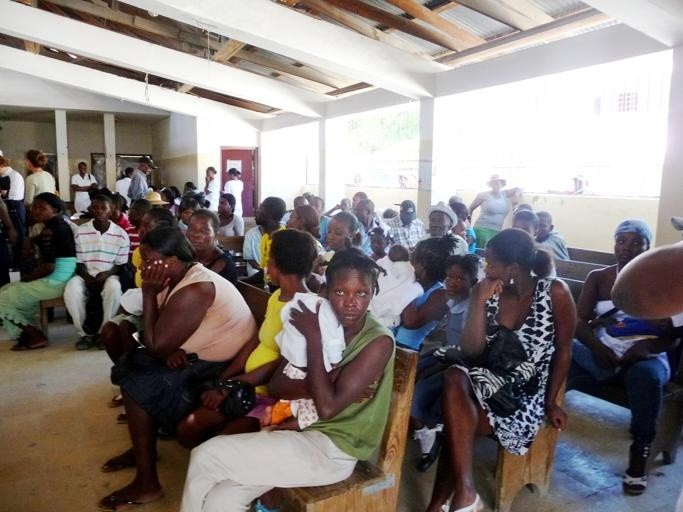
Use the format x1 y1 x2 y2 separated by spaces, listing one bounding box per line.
108 394 125 407
115 413 129 424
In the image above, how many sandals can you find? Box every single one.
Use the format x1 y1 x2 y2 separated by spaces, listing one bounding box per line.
438 492 483 512
621 471 646 495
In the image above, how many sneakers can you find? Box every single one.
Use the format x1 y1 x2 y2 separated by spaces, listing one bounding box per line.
75 336 95 349
414 424 447 474
11 334 49 350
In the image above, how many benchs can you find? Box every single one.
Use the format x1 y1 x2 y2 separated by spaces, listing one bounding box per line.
174 280 418 511
476 246 682 476
38 295 67 345
420 326 567 512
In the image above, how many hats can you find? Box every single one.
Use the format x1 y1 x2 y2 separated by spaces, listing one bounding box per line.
395 200 415 212
487 174 505 187
144 192 171 206
140 156 159 169
424 201 457 229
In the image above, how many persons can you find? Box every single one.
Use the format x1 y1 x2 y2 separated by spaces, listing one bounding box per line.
0 198 19 287
469 174 521 249
204 167 219 211
425 226 577 511
512 203 569 262
115 155 158 209
96 224 257 512
411 254 479 471
610 240 681 319
224 167 243 217
0 154 25 272
101 181 244 288
572 218 675 496
176 245 398 511
63 194 131 350
24 150 56 213
0 191 76 351
71 161 99 214
241 192 475 350
89 187 112 195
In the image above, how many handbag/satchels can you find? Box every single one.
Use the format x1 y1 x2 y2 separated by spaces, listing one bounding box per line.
191 374 256 419
19 235 55 282
433 326 539 417
